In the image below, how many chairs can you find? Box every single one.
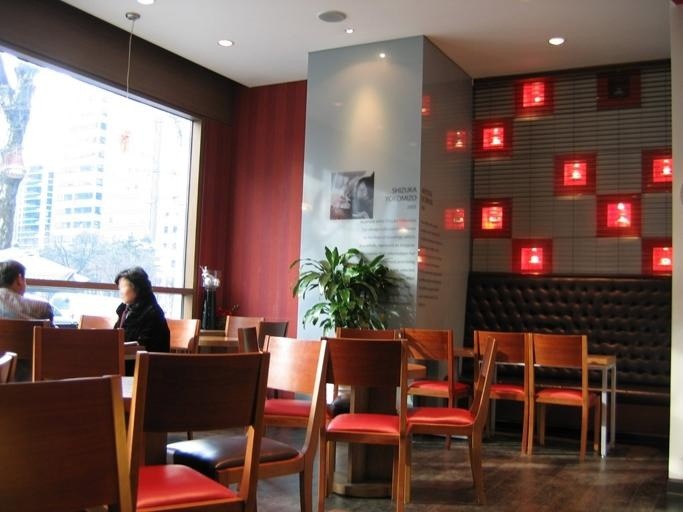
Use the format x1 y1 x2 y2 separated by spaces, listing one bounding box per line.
0 312 602 511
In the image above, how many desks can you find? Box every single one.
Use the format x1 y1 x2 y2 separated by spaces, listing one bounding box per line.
586 353 617 457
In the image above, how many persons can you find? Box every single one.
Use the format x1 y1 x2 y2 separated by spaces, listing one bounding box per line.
0 261 53 322
349 175 371 219
112 265 169 377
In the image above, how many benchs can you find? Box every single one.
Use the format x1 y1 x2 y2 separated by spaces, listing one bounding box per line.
442 270 670 445
